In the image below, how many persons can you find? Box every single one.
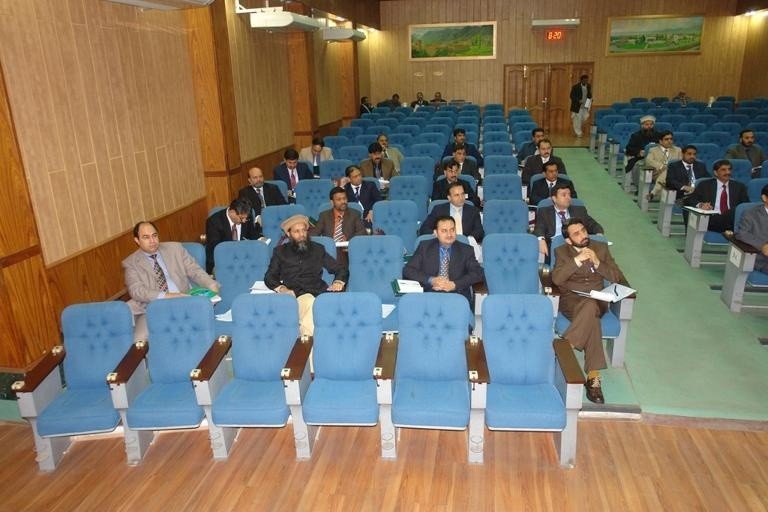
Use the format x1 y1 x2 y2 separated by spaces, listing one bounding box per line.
403 216 483 335
264 214 349 337
239 138 334 209
309 186 367 267
533 184 604 266
206 197 267 280
625 114 768 278
552 217 626 405
121 221 219 345
338 134 403 233
431 129 484 206
411 92 429 112
570 75 593 138
517 128 577 205
430 92 447 110
360 96 374 116
376 94 401 112
418 182 485 244
675 91 691 108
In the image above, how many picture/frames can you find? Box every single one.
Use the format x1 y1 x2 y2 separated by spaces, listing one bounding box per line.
409 21 497 62
606 14 705 56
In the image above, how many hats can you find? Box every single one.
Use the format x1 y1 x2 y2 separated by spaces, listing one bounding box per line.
640 115 656 124
280 215 309 232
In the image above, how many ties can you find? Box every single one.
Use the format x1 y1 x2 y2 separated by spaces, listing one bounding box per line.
256 189 263 208
334 213 343 241
548 183 553 194
687 166 693 177
383 150 388 158
664 150 667 156
720 185 728 214
355 187 360 199
375 165 380 177
291 170 296 186
313 156 317 166
232 224 237 240
453 206 463 235
149 254 168 294
440 246 449 277
559 212 566 223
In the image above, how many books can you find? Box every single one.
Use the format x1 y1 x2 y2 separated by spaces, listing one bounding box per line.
181 286 222 303
572 282 637 304
391 279 423 297
249 280 283 294
584 97 592 112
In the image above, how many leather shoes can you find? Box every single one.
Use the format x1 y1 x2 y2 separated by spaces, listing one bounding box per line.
647 192 653 202
585 377 604 403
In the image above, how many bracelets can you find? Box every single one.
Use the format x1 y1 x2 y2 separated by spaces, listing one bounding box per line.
538 237 546 240
